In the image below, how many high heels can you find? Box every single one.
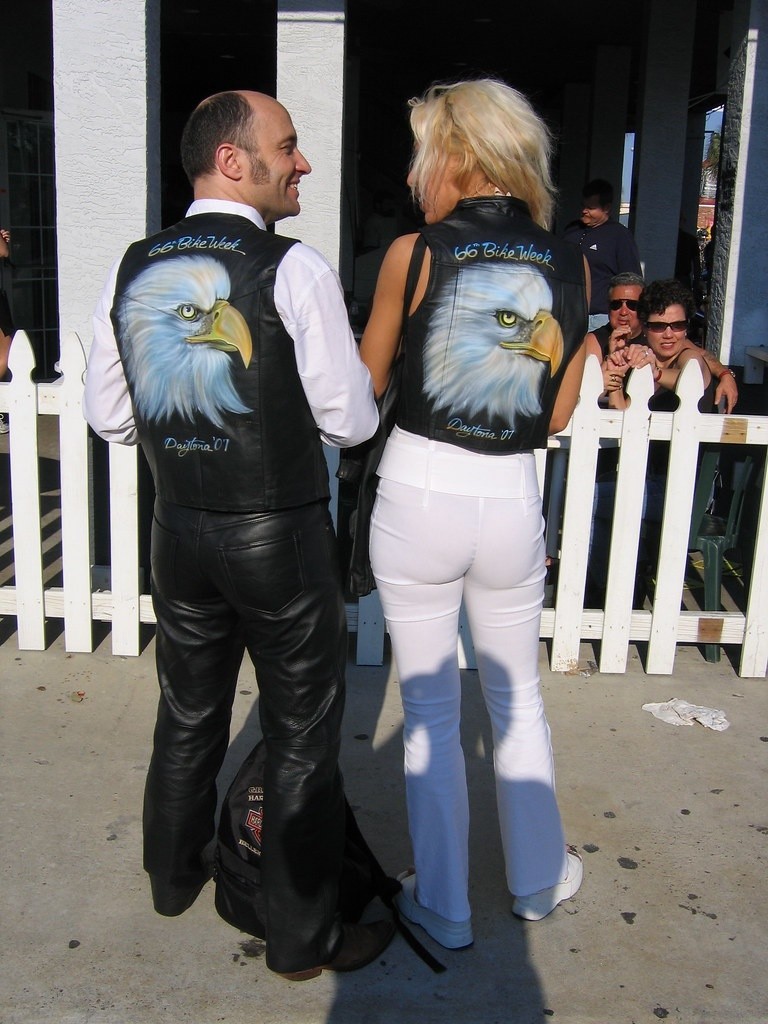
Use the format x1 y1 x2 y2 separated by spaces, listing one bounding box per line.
394 865 473 951
512 849 584 922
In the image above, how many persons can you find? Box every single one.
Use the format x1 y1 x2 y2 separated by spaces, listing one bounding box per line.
82 90 399 980
545 279 720 581
0 226 16 433
359 80 591 949
562 178 644 333
582 271 738 415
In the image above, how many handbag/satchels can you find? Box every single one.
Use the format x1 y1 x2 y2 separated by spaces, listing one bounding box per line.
334 362 404 602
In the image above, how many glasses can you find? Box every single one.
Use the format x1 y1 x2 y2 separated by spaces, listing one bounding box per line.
609 299 642 311
643 320 690 333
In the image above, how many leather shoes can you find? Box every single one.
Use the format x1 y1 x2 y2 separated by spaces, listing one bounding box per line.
150 873 213 917
276 918 397 981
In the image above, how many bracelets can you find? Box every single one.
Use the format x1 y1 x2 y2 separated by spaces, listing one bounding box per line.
654 367 662 382
719 369 736 379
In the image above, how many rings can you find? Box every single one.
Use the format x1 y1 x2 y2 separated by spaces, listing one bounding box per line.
643 350 648 357
612 374 617 382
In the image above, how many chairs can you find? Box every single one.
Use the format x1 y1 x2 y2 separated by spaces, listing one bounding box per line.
641 442 768 662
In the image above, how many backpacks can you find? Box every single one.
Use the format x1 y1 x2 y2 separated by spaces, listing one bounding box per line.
213 734 448 976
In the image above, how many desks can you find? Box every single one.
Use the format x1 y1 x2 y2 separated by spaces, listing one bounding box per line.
544 434 619 559
